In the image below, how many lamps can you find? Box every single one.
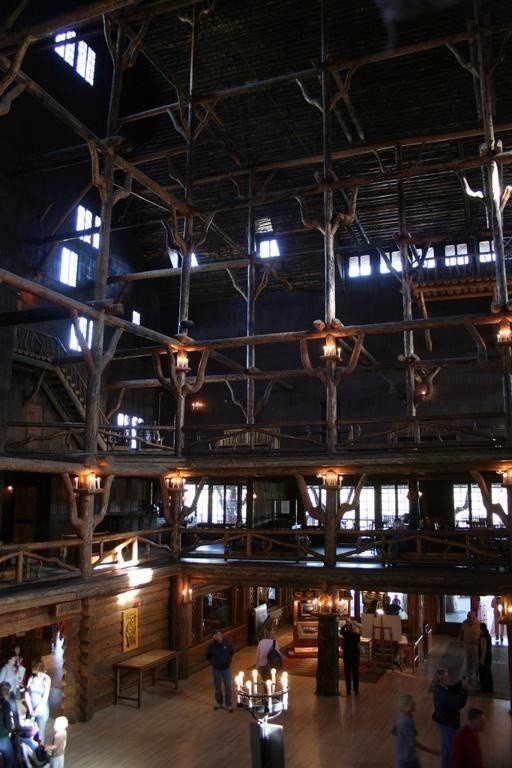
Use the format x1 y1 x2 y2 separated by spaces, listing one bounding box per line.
189 400 203 412
498 466 511 488
73 464 103 490
497 603 512 619
322 344 342 360
495 328 512 344
159 469 187 490
174 356 190 371
182 588 193 602
316 469 344 489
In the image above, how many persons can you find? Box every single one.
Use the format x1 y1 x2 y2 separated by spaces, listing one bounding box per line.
255 626 282 693
0 644 69 768
491 596 504 641
364 591 402 616
475 623 494 695
391 692 441 768
448 707 488 768
428 667 469 768
457 610 483 684
341 617 362 697
202 627 234 713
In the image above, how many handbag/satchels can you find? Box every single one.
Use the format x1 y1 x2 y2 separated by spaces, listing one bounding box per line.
391 724 397 733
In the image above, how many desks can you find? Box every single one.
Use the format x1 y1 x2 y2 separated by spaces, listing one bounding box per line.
398 633 424 674
113 648 184 709
359 636 372 667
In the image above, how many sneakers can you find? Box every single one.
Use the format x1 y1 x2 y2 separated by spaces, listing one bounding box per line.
213 702 233 712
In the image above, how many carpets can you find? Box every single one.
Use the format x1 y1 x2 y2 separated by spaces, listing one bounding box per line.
246 641 386 684
427 641 510 700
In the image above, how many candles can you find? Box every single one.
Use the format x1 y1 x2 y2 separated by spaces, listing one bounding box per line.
235 667 289 707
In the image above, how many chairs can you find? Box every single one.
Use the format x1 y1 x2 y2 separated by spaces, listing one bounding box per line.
371 521 387 555
213 427 282 450
387 420 462 445
466 522 503 560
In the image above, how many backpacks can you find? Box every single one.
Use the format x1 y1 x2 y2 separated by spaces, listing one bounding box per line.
267 639 281 670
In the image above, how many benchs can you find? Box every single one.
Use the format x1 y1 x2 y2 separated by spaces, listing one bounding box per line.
380 551 463 569
224 527 311 563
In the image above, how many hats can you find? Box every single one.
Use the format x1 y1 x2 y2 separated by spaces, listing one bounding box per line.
20 720 38 737
